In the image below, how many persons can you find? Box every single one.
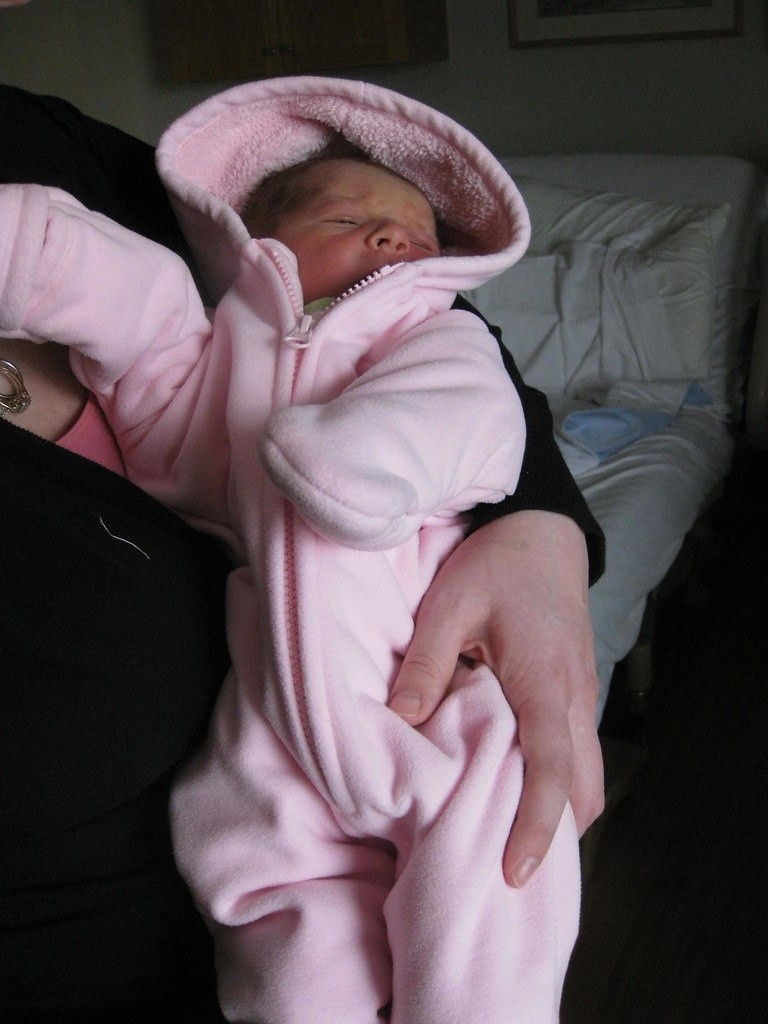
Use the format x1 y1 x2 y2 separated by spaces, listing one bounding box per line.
0 75 581 1024
0 67 607 1024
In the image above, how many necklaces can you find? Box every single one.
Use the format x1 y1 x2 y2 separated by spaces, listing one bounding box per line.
0 357 31 417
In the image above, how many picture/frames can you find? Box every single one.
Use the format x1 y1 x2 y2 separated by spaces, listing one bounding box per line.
506 0 744 49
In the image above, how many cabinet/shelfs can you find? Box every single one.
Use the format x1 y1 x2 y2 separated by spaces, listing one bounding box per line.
162 0 450 85
